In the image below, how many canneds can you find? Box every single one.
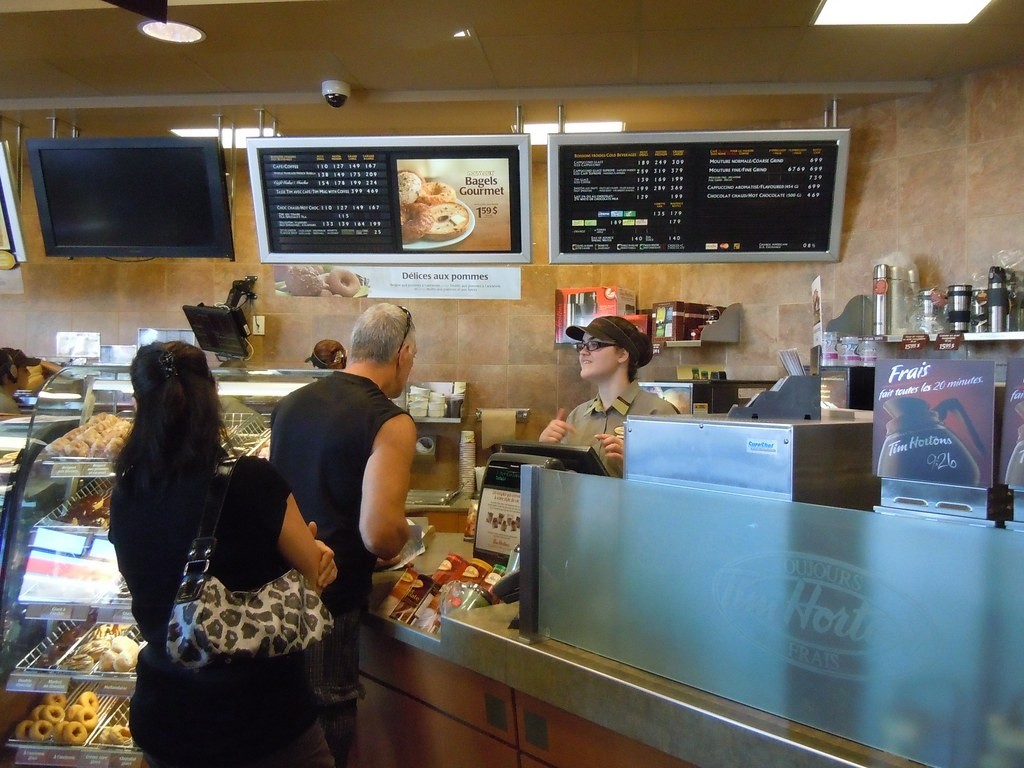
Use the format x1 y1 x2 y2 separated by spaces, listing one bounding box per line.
389 551 506 641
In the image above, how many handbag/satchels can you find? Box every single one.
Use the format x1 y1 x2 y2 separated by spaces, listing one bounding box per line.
165 458 336 670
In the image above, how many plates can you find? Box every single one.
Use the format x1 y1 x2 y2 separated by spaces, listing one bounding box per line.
402 200 475 251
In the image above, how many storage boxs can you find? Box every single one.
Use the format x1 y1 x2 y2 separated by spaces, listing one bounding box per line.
555 286 725 345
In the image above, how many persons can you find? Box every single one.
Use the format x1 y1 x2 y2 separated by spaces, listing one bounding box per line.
108 340 338 768
304 339 347 371
268 303 418 768
0 348 31 415
539 316 678 480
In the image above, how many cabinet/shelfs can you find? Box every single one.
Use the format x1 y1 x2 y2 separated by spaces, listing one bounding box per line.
0 361 324 768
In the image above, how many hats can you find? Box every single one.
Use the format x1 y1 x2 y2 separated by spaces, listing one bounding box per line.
566 317 646 362
0 349 42 374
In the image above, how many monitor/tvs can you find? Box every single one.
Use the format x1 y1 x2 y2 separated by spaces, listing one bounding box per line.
183 305 249 358
549 127 852 265
246 132 532 264
24 137 235 259
490 441 609 476
0 140 27 263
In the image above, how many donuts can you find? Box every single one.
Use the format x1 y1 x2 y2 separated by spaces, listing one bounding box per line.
13 691 132 747
53 494 113 528
398 169 469 244
34 622 128 671
329 268 360 298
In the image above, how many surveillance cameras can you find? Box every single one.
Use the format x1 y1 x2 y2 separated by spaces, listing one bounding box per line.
322 80 351 108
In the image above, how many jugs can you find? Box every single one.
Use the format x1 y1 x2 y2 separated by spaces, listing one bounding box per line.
878 396 986 487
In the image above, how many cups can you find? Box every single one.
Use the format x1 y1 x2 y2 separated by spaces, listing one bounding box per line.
861 341 879 367
822 332 838 367
446 394 464 418
694 403 708 416
439 581 494 625
409 386 446 418
839 336 860 367
458 431 476 498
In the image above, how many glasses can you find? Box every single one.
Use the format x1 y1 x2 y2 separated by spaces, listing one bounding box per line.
572 341 616 352
398 304 411 352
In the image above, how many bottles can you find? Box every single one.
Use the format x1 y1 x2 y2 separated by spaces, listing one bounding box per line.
947 285 971 334
988 266 1007 332
718 372 727 381
972 289 988 333
701 371 709 380
889 266 904 335
906 270 917 294
872 264 888 336
918 290 938 335
692 367 699 380
711 371 718 380
1006 268 1016 331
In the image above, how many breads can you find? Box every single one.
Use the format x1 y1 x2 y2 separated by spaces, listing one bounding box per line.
44 412 134 460
285 265 324 297
0 452 20 466
99 634 143 672
25 361 66 391
